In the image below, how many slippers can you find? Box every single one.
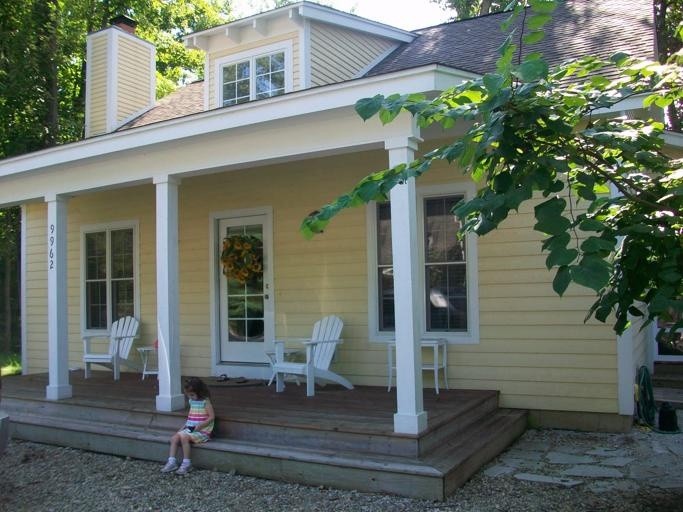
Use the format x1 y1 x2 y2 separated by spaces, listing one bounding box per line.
236 377 248 383
217 374 230 381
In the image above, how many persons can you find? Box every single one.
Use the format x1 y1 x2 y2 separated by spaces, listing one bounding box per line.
160 377 215 475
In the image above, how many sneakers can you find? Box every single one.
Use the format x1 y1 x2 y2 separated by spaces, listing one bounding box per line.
159 462 194 475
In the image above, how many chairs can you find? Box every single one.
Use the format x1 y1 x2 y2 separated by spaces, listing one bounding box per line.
81 315 140 382
273 315 355 396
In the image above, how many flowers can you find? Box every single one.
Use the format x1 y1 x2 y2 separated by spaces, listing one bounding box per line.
219 236 264 286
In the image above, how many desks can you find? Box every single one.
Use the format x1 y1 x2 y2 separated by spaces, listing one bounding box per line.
264 349 300 388
385 338 449 395
137 346 160 380
